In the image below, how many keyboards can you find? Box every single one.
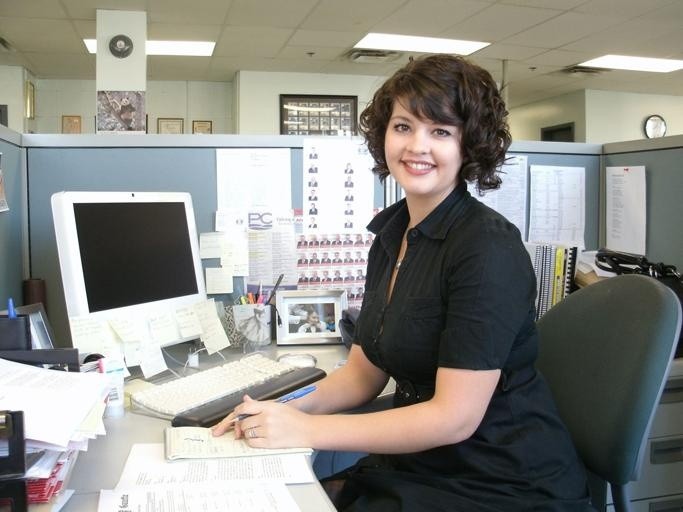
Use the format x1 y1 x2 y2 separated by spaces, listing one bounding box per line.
129 353 297 419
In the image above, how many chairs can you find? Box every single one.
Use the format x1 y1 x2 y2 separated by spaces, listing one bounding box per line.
527 271 683 511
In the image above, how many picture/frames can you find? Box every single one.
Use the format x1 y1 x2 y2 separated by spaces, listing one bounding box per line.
274 288 348 346
61 115 213 134
279 93 359 136
25 79 36 120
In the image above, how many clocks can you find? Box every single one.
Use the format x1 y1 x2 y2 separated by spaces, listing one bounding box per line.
643 114 666 140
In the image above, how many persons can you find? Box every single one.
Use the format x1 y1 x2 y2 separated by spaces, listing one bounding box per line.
207 48 595 511
298 232 375 304
289 303 335 334
341 163 356 231
306 145 319 231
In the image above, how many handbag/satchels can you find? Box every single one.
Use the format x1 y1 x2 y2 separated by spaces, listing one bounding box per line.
595 248 683 359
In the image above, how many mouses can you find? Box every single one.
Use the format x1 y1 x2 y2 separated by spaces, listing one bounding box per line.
277 352 316 369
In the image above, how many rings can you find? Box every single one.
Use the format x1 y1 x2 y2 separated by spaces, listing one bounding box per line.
246 428 257 438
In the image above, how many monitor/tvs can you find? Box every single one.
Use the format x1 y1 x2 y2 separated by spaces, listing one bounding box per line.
51 190 209 381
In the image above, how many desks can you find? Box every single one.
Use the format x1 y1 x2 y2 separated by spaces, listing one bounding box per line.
0 306 400 511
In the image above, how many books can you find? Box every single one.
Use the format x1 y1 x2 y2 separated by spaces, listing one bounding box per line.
521 239 579 329
127 352 327 429
0 353 138 507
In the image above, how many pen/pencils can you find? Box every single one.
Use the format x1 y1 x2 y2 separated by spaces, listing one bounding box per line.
236 274 285 305
228 386 317 424
7 297 16 321
98 358 104 373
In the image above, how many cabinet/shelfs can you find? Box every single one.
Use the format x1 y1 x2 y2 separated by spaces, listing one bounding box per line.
607 359 683 511
